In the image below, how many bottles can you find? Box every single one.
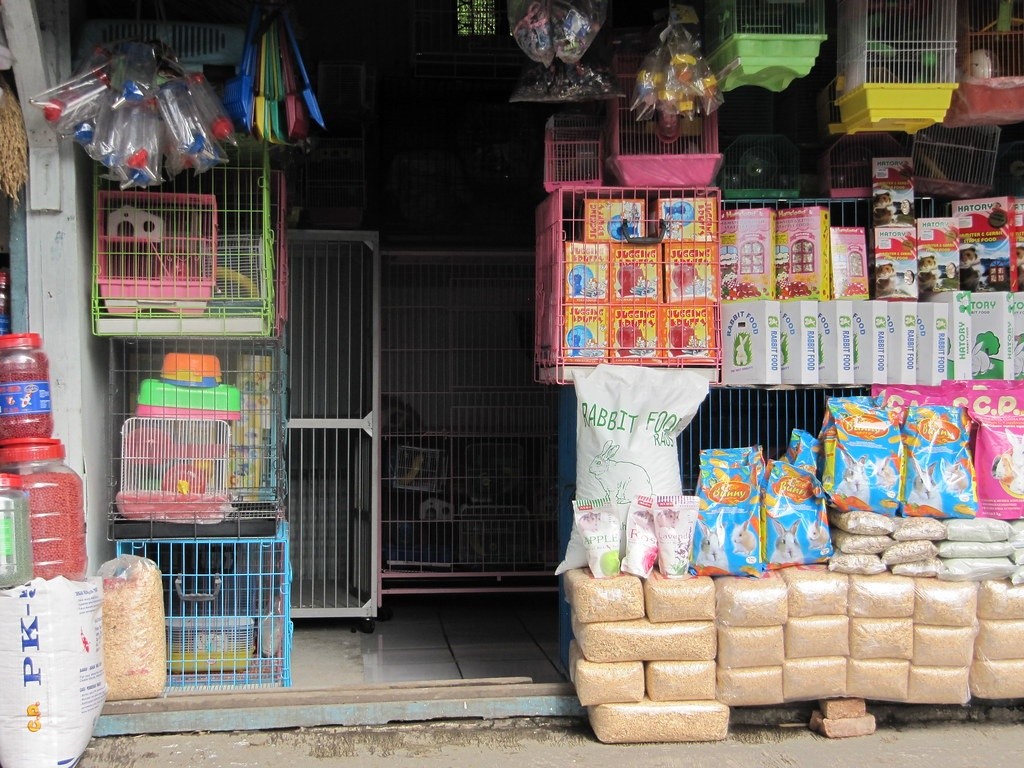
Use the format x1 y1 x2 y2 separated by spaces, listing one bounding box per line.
0 333 53 439
0 473 34 588
0 272 10 334
0 438 88 580
29 45 240 191
